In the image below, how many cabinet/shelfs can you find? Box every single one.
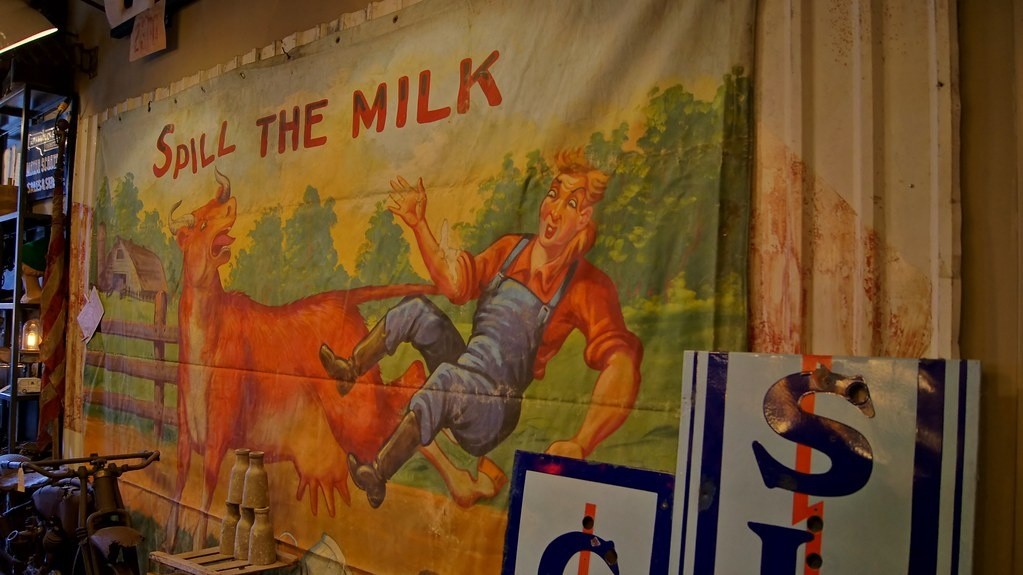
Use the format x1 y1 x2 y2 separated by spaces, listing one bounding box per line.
0 79 81 454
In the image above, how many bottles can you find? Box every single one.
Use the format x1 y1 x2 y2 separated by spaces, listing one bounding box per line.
234 504 253 560
219 501 240 555
242 451 270 508
227 448 251 503
248 507 276 565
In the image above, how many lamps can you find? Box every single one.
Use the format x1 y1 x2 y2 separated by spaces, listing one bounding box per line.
19 319 43 353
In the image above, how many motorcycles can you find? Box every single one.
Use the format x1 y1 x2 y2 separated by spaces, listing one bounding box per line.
0 449 162 575
